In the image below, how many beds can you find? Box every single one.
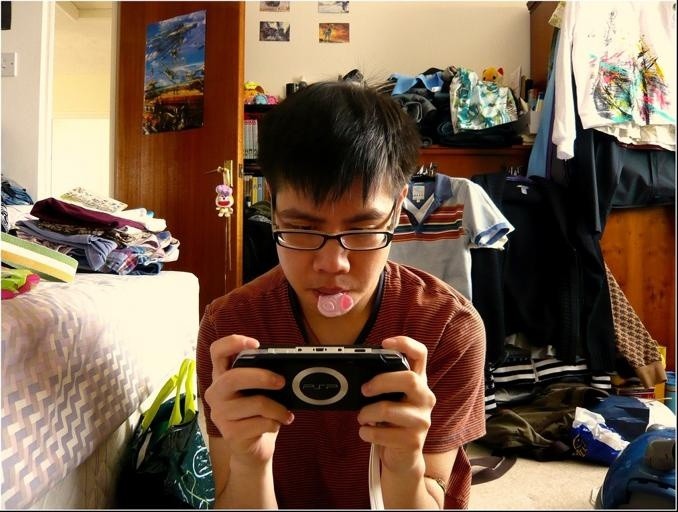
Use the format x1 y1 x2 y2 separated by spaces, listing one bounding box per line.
2 270 200 508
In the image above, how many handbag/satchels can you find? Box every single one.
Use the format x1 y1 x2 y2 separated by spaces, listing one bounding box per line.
472 380 609 460
120 358 215 510
570 406 631 465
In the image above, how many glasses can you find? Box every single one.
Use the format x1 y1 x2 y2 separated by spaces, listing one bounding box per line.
270 192 398 252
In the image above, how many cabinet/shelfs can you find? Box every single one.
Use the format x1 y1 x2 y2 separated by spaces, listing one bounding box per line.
526 2 676 373
242 101 281 214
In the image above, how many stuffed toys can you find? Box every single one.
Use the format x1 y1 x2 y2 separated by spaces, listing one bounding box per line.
244 80 278 105
214 183 235 219
482 66 505 83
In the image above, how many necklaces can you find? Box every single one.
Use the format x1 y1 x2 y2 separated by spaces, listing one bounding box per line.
304 314 322 345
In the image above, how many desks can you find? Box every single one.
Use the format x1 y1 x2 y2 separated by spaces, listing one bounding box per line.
413 145 524 179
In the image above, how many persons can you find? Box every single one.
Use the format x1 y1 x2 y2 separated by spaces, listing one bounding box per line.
322 23 332 41
194 77 489 509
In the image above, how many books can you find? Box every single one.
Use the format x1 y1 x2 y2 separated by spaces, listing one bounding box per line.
243 118 272 211
